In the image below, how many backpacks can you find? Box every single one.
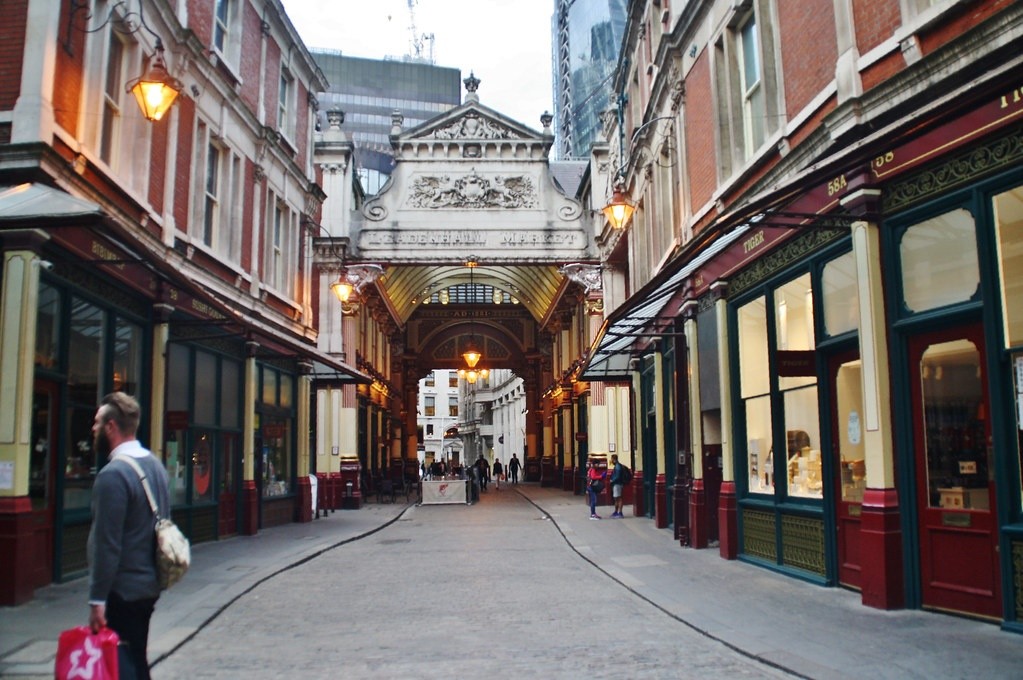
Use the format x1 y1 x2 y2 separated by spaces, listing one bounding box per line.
617 462 631 484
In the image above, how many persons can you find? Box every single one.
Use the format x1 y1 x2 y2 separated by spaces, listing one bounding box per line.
493 458 503 490
508 454 522 485
586 459 607 520
421 458 464 480
472 454 490 492
85 392 171 680
609 455 625 518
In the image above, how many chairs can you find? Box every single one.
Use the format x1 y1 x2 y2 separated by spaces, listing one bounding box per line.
395 480 413 504
362 481 378 503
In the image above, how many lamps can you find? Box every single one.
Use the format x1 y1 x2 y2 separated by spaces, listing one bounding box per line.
602 116 677 233
422 290 431 305
510 290 520 304
300 219 355 302
438 289 450 305
462 258 482 369
492 288 503 304
456 369 491 384
62 0 185 122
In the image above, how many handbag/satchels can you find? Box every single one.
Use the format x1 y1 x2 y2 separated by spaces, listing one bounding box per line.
501 474 504 480
54 628 120 680
508 471 511 478
590 479 605 494
110 454 191 591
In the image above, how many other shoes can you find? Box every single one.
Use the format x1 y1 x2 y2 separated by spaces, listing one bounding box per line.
610 511 623 518
590 514 601 520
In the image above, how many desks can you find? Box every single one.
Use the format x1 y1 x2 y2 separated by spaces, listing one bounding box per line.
421 476 468 504
